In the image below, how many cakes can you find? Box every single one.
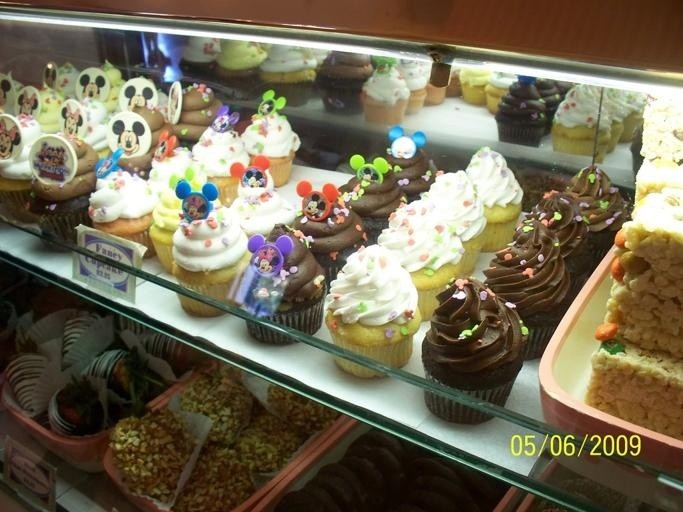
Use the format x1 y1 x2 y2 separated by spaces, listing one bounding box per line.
172 179 252 316
313 48 375 118
227 222 328 343
14 79 68 134
566 164 633 263
147 148 209 275
240 89 301 187
459 66 493 106
323 244 421 380
179 37 221 76
75 60 124 106
394 50 434 118
88 149 156 261
361 61 410 128
229 156 296 251
530 190 597 305
283 181 370 293
622 93 646 143
376 202 464 324
496 81 544 150
606 86 624 154
422 277 530 425
118 74 170 119
0 74 27 114
551 85 611 169
484 75 524 116
28 132 99 253
463 146 523 255
44 62 82 102
335 155 408 251
192 105 250 216
425 47 455 108
596 89 624 155
484 221 577 363
260 43 317 106
383 126 440 206
532 77 563 132
58 99 110 151
168 78 229 145
0 112 45 223
420 168 488 280
214 38 266 100
106 102 176 180
